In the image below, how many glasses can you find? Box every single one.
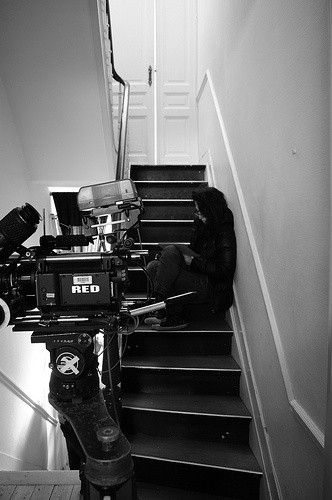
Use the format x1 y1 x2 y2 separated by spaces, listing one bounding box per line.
193 209 202 216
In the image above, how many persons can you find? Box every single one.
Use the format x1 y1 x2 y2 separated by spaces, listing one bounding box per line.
57 337 108 470
144 186 238 331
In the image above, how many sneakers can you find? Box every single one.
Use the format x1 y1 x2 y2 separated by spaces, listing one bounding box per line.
153 314 189 332
145 309 167 324
